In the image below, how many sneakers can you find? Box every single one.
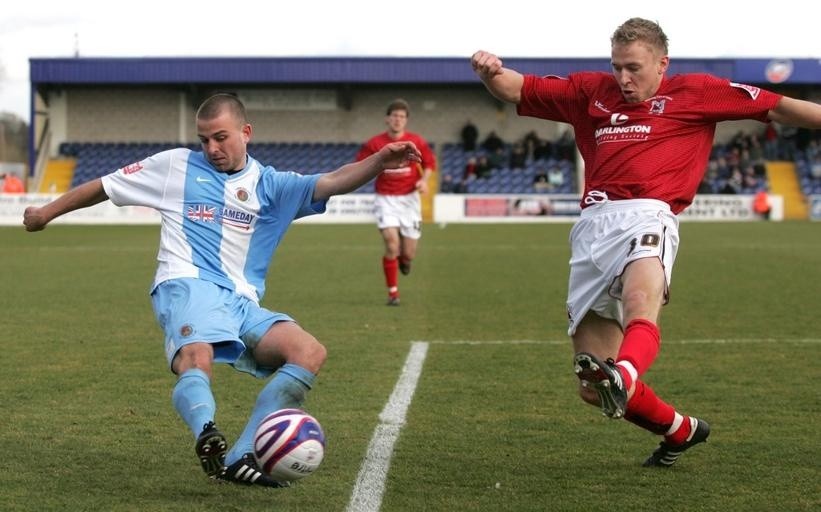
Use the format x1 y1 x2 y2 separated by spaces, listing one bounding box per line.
214 455 292 488
196 423 228 481
644 415 712 469
574 351 629 421
385 255 411 307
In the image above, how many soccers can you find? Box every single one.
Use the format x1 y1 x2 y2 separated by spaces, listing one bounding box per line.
252 408 325 481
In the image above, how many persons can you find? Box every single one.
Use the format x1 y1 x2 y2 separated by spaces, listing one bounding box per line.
471 16 821 471
23 92 424 489
751 187 772 222
353 95 436 308
440 115 580 193
696 124 820 194
1 172 25 193
